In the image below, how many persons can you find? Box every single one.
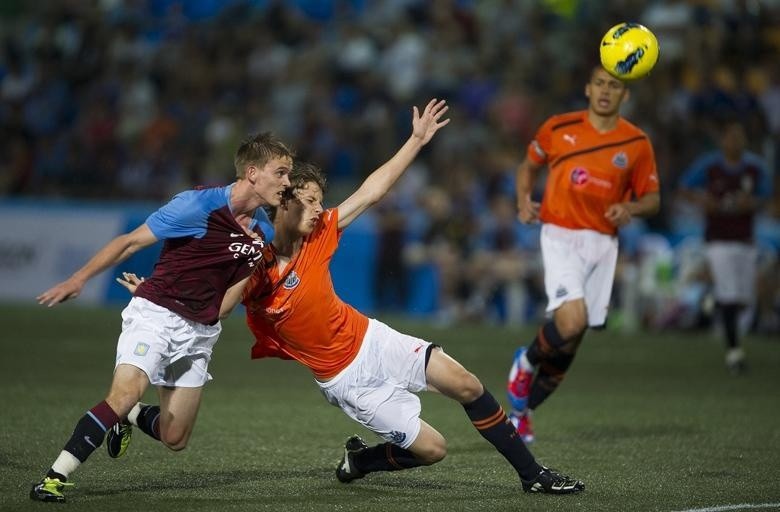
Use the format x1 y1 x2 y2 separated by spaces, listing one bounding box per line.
507 64 661 445
678 123 773 374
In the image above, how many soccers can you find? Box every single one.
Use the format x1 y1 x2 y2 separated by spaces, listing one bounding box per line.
600 22 659 81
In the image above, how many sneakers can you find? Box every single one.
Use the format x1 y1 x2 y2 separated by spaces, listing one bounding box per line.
29 468 67 503
520 464 585 494
107 415 133 458
336 434 368 484
507 347 536 444
